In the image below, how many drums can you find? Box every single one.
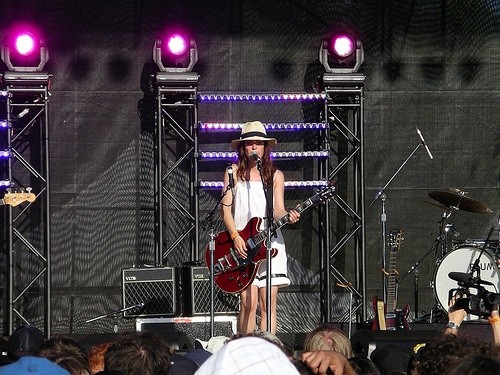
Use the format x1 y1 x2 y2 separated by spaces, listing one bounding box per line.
451 238 499 250
429 243 499 324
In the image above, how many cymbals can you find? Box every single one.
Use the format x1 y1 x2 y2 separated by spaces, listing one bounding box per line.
427 190 493 214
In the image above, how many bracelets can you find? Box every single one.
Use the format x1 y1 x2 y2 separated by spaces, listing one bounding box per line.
231 231 239 240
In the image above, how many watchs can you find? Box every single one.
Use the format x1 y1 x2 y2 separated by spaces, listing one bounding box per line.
446 322 458 330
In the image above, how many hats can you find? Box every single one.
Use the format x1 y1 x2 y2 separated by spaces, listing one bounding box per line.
370 344 414 372
0 356 72 375
231 121 277 150
164 331 301 375
7 325 44 360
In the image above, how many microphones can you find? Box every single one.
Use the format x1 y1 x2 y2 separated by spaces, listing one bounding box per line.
415 127 433 159
448 272 494 286
250 152 258 160
228 162 234 198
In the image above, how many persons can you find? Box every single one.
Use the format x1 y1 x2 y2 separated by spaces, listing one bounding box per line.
0 309 500 375
222 120 301 334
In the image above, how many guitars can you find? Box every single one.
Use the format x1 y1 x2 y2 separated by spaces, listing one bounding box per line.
0 186 36 208
372 228 412 331
206 184 337 294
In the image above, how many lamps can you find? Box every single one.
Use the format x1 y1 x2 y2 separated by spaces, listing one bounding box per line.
319 28 365 75
153 26 198 73
1 29 48 72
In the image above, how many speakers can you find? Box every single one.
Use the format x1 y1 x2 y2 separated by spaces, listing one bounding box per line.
351 329 443 363
121 267 177 318
190 266 241 317
